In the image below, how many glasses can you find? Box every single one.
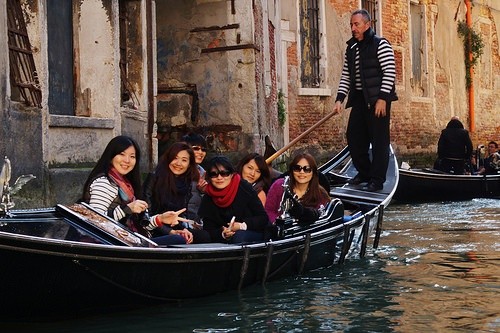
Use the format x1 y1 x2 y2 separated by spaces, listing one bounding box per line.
292 165 314 173
209 170 231 178
192 147 207 152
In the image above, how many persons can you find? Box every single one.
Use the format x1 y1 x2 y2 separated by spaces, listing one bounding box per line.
433 116 473 175
333 10 398 191
64 135 331 247
470 141 500 175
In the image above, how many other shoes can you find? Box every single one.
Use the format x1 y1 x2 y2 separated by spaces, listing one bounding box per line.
362 180 383 192
349 173 369 185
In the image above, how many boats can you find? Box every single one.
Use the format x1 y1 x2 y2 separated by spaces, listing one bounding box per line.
0 144 399 310
392 167 500 204
